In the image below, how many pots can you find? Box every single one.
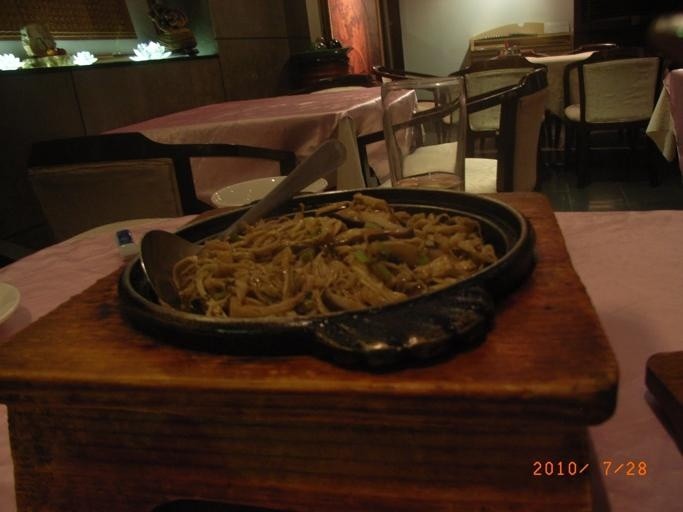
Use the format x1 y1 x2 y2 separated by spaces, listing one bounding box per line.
116 182 536 370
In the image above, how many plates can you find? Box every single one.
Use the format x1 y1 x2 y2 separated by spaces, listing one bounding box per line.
211 175 329 210
291 46 353 67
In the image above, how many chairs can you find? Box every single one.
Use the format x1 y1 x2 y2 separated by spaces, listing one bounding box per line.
353 65 548 195
12 131 298 244
559 46 669 198
372 64 441 83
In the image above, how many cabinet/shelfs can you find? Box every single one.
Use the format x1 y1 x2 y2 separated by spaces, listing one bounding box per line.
1 54 226 151
